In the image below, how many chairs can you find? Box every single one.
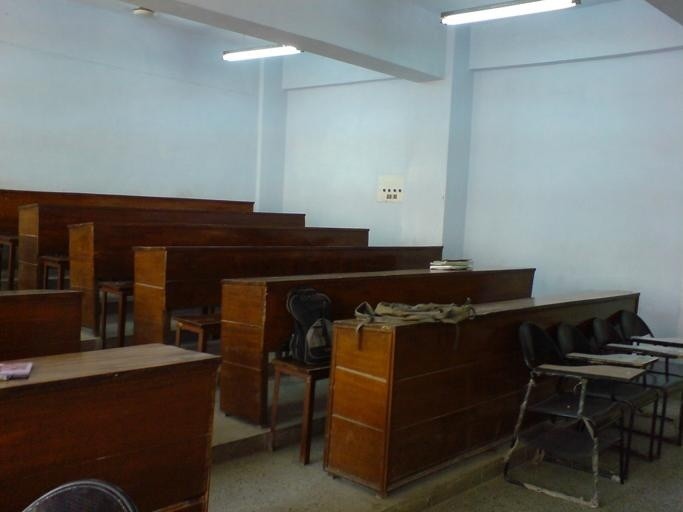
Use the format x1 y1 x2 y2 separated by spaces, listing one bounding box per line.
499 310 682 507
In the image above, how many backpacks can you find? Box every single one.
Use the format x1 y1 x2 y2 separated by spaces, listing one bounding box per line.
287 288 334 364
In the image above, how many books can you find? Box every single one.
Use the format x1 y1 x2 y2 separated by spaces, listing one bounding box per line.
0 360 32 380
429 259 468 270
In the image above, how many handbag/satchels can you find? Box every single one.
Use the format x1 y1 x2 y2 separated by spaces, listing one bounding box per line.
356 297 473 332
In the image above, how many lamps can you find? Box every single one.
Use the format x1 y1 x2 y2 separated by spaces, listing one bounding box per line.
133 6 153 18
221 45 304 63
440 0 583 26
